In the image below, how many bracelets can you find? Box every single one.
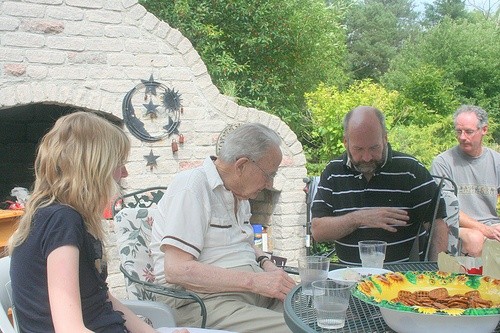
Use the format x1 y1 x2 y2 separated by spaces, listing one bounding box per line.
257 256 271 269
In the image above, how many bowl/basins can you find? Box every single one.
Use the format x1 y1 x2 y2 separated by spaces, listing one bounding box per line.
350 270 500 333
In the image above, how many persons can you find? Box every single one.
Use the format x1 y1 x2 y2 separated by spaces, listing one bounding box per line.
431 105 500 256
9 111 190 333
150 123 298 333
310 106 448 266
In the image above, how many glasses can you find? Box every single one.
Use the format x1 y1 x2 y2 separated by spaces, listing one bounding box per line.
237 157 278 185
453 125 484 139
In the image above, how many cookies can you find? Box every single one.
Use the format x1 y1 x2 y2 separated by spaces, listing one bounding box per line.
391 287 494 310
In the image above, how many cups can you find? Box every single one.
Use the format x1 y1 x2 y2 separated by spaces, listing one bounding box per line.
481 236 500 281
357 240 387 268
298 255 331 296
311 279 354 330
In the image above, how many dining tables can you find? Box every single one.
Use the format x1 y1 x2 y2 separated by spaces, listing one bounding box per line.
283 260 500 333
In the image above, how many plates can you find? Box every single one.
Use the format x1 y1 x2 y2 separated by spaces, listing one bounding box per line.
327 267 395 286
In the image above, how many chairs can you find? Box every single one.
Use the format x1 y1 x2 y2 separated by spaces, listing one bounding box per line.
303 176 460 264
0 255 176 333
112 187 300 329
